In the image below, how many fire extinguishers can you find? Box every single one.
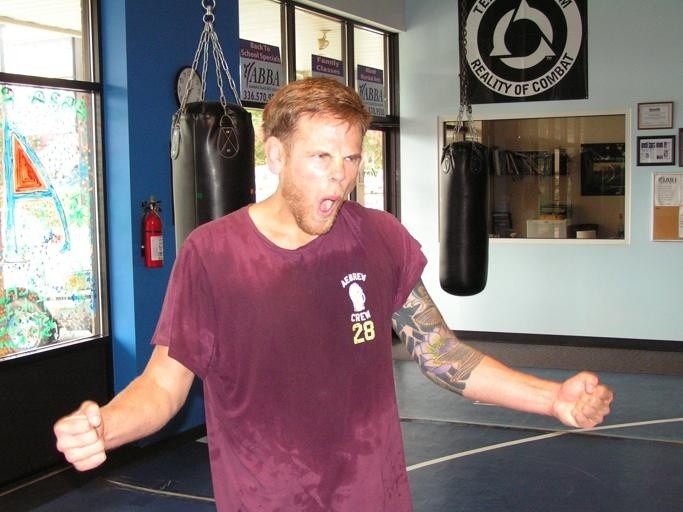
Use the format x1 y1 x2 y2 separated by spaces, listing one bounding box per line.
141 195 164 268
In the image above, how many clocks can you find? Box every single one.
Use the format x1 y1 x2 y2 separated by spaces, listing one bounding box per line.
173 65 203 108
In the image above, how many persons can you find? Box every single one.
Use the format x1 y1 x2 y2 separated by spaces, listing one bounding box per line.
52 77 616 512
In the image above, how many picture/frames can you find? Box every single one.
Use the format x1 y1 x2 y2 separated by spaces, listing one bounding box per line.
636 101 675 166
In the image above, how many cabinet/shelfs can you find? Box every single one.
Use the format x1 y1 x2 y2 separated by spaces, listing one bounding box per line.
526 219 572 239
